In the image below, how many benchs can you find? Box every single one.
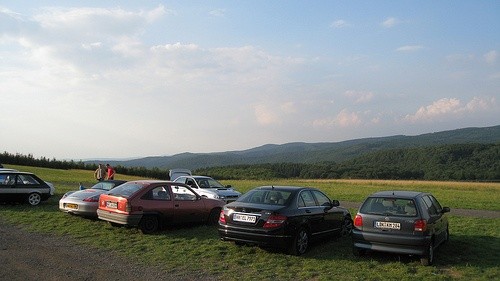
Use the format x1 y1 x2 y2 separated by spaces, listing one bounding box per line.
369 202 416 215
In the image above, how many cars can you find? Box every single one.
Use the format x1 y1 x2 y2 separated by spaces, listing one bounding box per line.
59 180 128 218
0 171 51 207
218 185 354 257
162 169 242 203
97 180 226 235
0 169 56 197
352 190 451 267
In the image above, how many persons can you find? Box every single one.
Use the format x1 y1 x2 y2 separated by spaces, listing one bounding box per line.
93 164 107 183
106 164 115 180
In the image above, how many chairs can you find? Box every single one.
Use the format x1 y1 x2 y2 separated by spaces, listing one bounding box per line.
158 191 165 198
200 181 206 185
270 192 283 204
187 181 193 185
5 176 12 185
383 200 399 214
